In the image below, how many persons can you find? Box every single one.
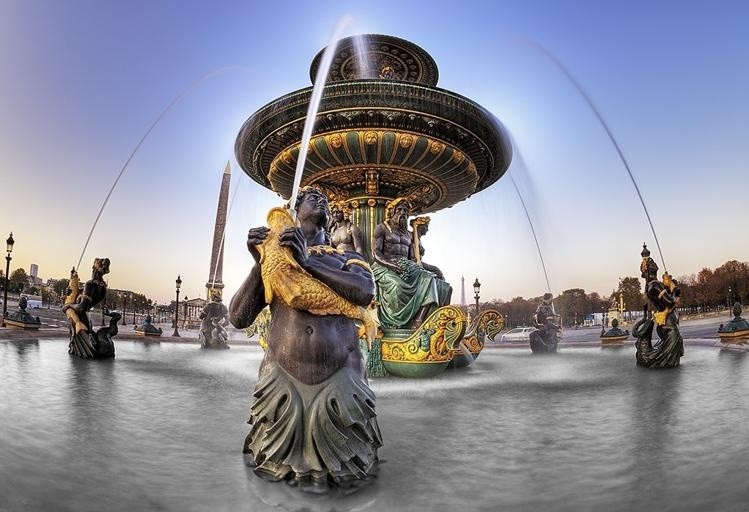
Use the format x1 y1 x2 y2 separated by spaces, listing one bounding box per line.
640 256 684 368
62 258 111 358
199 288 230 350
370 197 453 330
529 292 562 352
328 200 363 259
229 186 384 495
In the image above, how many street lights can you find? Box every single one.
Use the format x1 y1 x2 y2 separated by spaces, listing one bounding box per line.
172 275 183 336
184 296 188 328
3 232 14 316
133 298 136 324
473 277 480 315
728 286 732 318
601 306 605 332
141 300 167 323
123 292 126 325
